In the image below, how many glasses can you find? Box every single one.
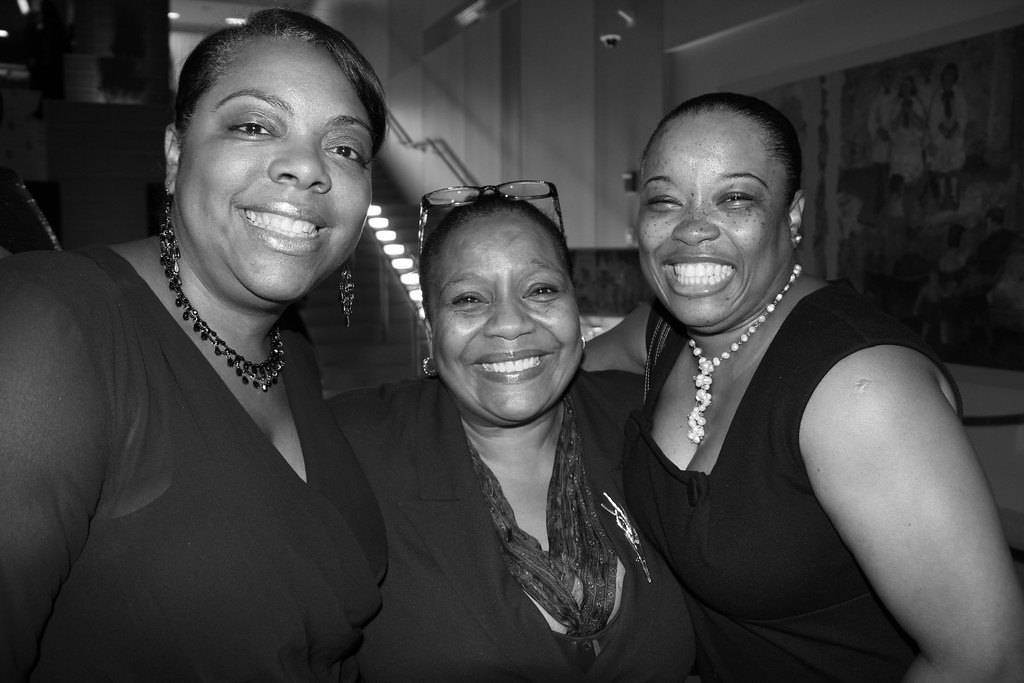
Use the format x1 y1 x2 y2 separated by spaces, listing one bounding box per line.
419 180 567 263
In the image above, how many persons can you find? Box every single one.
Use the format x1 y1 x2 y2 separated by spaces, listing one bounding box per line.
831 54 1012 362
325 191 698 683
580 91 1024 683
0 11 389 683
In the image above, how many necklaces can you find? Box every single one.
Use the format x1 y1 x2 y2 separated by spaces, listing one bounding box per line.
159 207 286 393
687 263 804 444
